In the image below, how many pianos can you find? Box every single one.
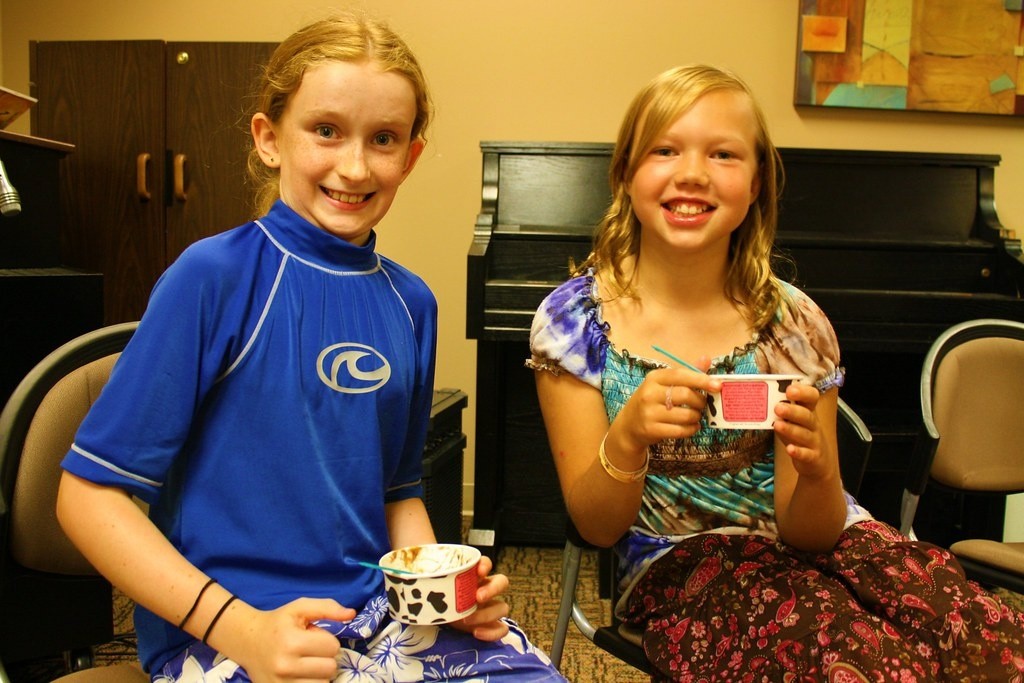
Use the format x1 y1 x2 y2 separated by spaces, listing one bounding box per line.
463 139 1022 544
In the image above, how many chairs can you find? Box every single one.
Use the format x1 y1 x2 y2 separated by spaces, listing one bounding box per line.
896 319 1023 595
548 393 871 683
0 322 151 683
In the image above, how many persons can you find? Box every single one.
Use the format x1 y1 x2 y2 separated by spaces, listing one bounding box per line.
524 65 1024 683
55 12 569 683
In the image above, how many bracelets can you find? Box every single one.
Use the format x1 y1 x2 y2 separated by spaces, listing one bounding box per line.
178 578 216 630
599 432 649 482
201 594 238 648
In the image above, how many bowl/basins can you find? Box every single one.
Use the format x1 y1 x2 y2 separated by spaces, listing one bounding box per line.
703 374 803 430
378 543 481 625
